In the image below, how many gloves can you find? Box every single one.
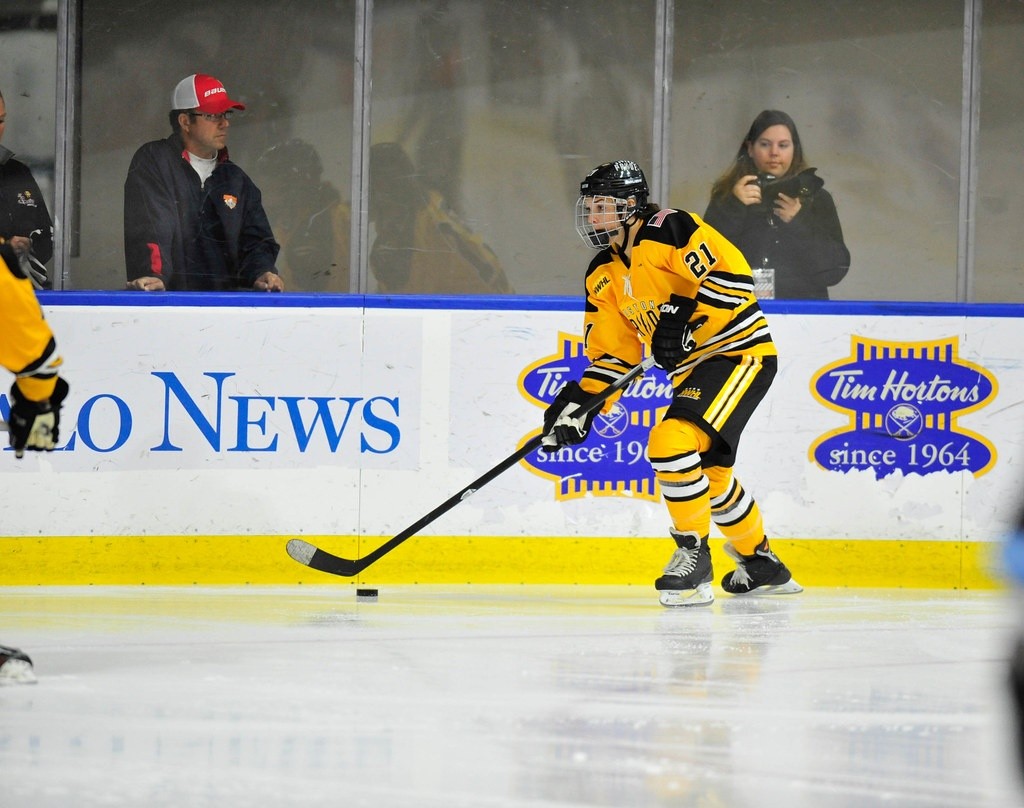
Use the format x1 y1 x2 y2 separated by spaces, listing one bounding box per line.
19 251 47 290
8 376 69 459
651 300 696 369
541 379 606 452
30 226 54 264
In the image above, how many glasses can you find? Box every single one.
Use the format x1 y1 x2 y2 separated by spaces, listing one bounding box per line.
185 109 235 123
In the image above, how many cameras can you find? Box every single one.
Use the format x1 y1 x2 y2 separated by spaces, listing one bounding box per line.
747 166 824 214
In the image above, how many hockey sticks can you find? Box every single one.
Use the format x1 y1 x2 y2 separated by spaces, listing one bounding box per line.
284 338 693 578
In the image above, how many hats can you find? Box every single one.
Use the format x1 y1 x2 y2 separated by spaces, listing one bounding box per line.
171 73 247 115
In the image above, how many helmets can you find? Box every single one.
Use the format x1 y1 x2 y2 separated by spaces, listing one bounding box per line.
580 161 650 198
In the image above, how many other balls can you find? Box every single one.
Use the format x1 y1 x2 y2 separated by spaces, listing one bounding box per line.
357 587 379 598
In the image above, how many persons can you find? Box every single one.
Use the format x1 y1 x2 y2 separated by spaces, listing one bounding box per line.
542 159 803 606
0 235 68 458
703 110 850 300
124 74 284 294
254 0 510 294
0 92 54 290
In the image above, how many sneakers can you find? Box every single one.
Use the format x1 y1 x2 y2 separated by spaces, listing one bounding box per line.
0 646 39 688
655 527 715 608
721 535 804 596
